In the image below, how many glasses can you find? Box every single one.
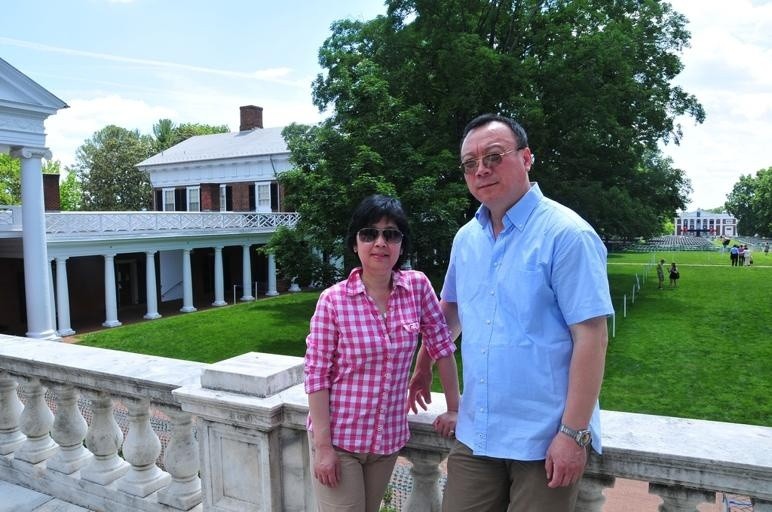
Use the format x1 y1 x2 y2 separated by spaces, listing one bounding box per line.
458 146 522 175
357 227 404 245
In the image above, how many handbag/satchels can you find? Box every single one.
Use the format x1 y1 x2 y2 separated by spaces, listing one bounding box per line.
669 272 679 279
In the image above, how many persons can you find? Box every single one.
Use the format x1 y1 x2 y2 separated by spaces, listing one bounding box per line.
302 192 463 510
666 262 677 288
719 238 769 267
405 113 615 512
656 259 665 289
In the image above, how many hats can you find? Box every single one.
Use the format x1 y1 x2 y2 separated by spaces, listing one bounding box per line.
659 259 666 263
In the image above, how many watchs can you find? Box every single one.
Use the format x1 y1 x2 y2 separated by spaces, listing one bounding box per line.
557 423 593 450
